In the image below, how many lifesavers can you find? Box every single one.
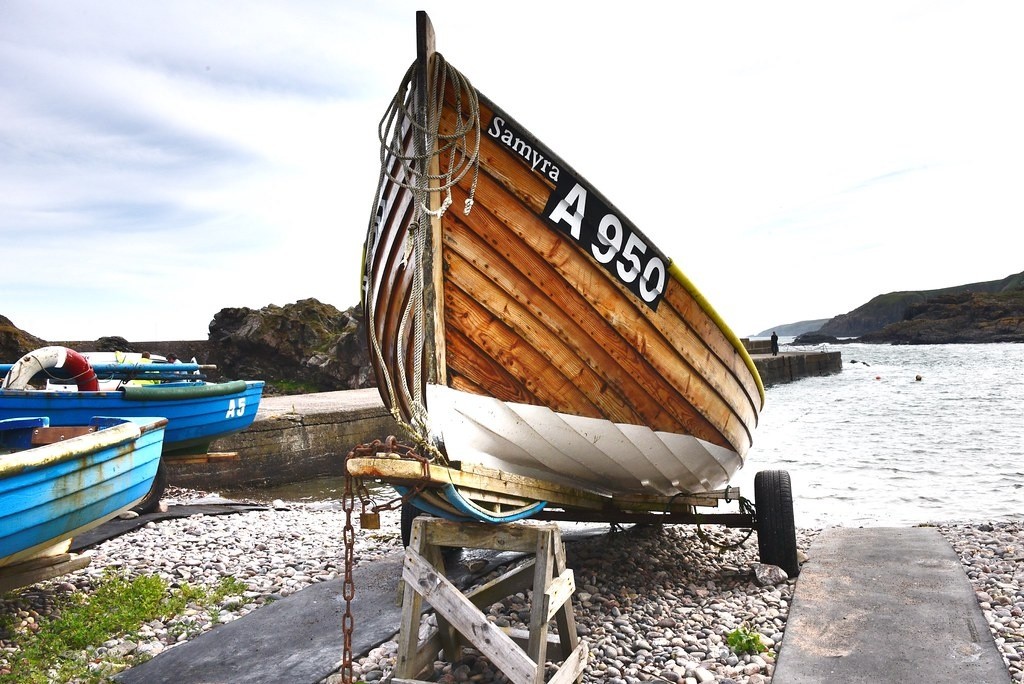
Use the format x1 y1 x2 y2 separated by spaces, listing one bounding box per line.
2 345 100 391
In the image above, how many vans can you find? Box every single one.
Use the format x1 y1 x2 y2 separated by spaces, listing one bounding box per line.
45 350 168 391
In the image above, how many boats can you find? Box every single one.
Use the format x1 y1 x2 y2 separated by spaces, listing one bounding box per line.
0 414 168 567
0 363 267 442
358 9 766 498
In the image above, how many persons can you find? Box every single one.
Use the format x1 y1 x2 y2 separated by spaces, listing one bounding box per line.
165 352 187 383
771 332 778 356
131 351 160 387
